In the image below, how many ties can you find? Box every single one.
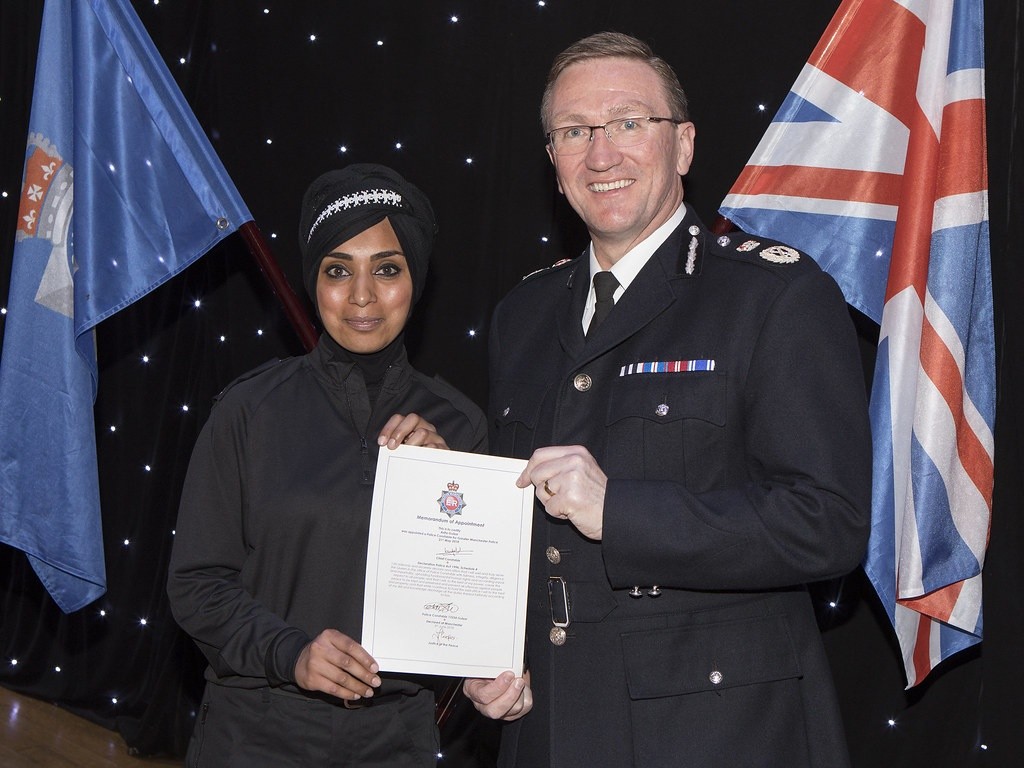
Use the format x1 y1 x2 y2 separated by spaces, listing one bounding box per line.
586 272 621 338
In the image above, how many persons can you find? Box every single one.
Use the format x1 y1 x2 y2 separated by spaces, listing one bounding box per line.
464 32 870 768
165 164 490 768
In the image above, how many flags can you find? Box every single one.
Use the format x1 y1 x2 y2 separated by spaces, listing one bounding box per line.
718 0 996 691
0 0 253 613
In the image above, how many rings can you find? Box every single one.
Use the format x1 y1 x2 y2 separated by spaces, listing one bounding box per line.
541 480 556 497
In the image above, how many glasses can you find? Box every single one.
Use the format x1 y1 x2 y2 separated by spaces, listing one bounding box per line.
547 117 678 156
